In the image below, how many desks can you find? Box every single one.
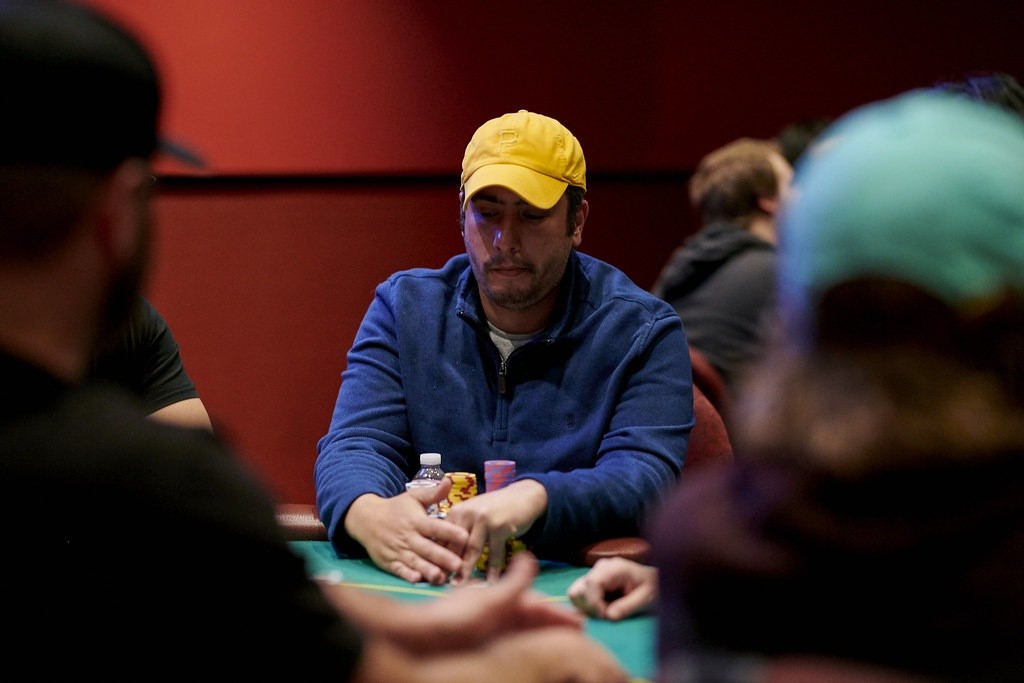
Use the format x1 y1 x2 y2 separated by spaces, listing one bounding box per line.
267 507 657 683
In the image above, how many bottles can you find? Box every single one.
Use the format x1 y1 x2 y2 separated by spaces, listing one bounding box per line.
411 453 448 517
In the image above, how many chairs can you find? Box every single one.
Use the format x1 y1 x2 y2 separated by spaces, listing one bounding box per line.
683 344 738 480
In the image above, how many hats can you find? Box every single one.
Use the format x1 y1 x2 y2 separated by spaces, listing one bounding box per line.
0 0 205 174
776 87 1024 340
460 109 588 211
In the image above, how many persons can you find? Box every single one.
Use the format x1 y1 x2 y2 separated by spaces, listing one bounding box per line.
569 72 1024 623
315 109 696 583
0 0 626 682
649 93 1024 683
651 140 793 385
